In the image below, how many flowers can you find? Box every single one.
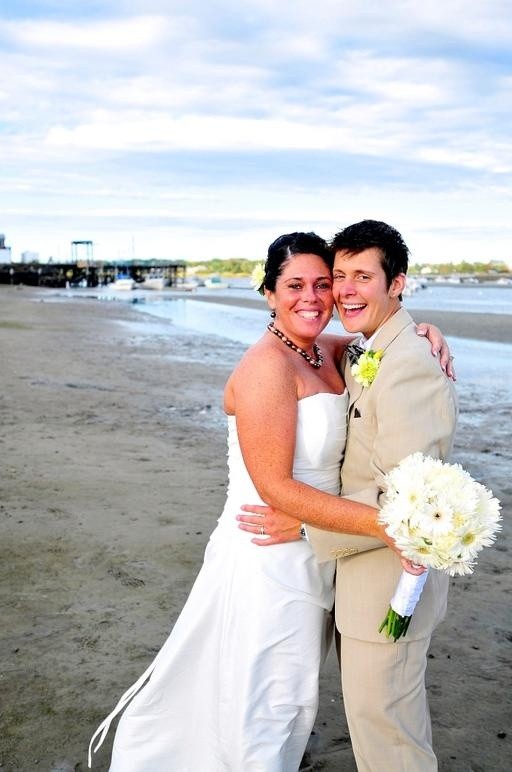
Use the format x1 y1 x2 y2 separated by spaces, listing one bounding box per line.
250 264 266 296
377 452 503 643
349 349 383 387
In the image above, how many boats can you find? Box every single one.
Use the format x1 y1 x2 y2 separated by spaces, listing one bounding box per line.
204 277 230 289
176 282 198 291
106 277 136 290
141 273 170 291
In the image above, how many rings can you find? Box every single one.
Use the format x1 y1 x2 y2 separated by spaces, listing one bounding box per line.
259 526 267 535
449 354 456 364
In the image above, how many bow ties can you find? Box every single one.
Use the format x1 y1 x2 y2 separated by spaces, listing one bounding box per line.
344 343 365 366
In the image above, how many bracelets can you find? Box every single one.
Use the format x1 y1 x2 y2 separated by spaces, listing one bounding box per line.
298 522 308 541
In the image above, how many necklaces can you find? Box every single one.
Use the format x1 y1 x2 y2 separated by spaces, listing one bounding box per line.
265 320 327 371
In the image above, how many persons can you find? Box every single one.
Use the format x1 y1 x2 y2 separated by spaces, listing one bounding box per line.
233 218 459 772
87 229 460 772
